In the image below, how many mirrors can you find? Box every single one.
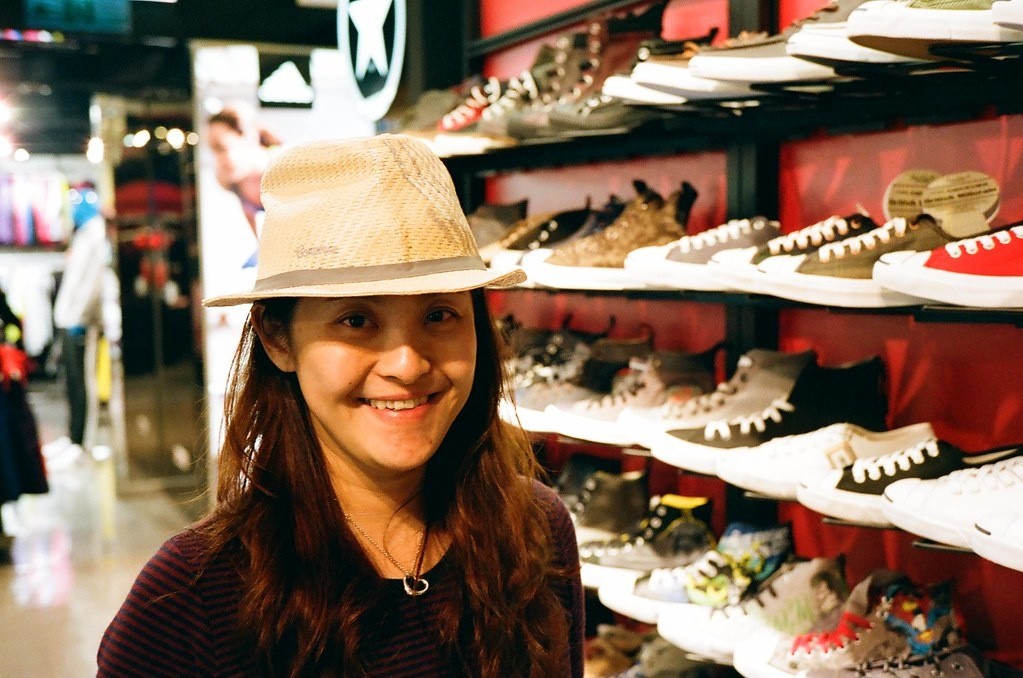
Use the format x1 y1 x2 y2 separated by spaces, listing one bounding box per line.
92 94 203 498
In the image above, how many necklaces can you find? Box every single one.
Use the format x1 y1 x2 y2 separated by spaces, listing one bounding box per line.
333 497 430 596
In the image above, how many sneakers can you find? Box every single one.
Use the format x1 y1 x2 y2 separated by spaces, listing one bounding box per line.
406 0 1022 678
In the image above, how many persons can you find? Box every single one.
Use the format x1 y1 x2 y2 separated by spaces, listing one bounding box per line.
204 107 287 247
40 180 109 473
94 132 585 678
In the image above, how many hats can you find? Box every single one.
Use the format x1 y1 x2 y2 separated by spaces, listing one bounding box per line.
200 135 527 308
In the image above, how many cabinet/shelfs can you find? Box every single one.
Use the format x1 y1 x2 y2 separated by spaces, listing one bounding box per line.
397 0 1023 678
